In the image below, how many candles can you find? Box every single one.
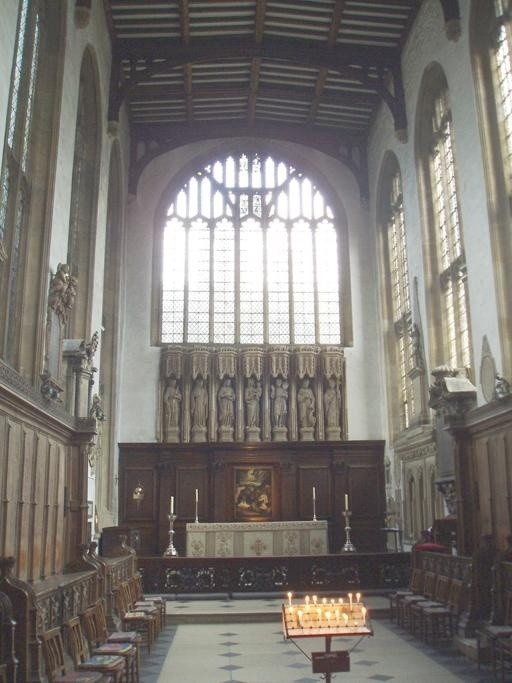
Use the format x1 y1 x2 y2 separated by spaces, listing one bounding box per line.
287 591 366 627
344 494 349 511
195 488 199 502
170 496 175 515
312 487 315 500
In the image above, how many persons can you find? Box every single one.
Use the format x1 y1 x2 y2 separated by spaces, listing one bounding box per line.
50 265 70 297
164 378 341 428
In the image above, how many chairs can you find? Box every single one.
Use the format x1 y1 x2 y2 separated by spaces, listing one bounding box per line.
475 590 512 683
388 567 465 651
112 573 167 655
37 600 141 683
